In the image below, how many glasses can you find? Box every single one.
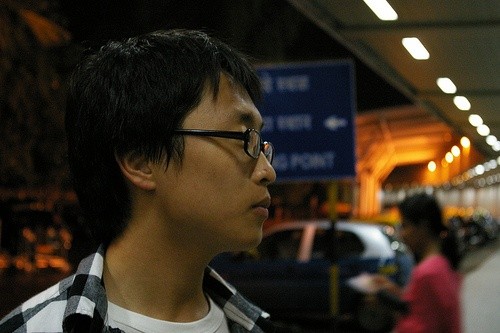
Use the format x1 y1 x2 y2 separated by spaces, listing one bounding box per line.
174 128 274 166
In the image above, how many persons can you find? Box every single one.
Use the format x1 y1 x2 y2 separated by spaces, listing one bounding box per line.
1 28 277 333
372 194 462 333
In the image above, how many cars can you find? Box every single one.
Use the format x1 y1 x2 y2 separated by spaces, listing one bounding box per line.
207 220 413 311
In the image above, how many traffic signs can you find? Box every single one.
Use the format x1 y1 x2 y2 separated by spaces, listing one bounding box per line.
254 62 358 182
